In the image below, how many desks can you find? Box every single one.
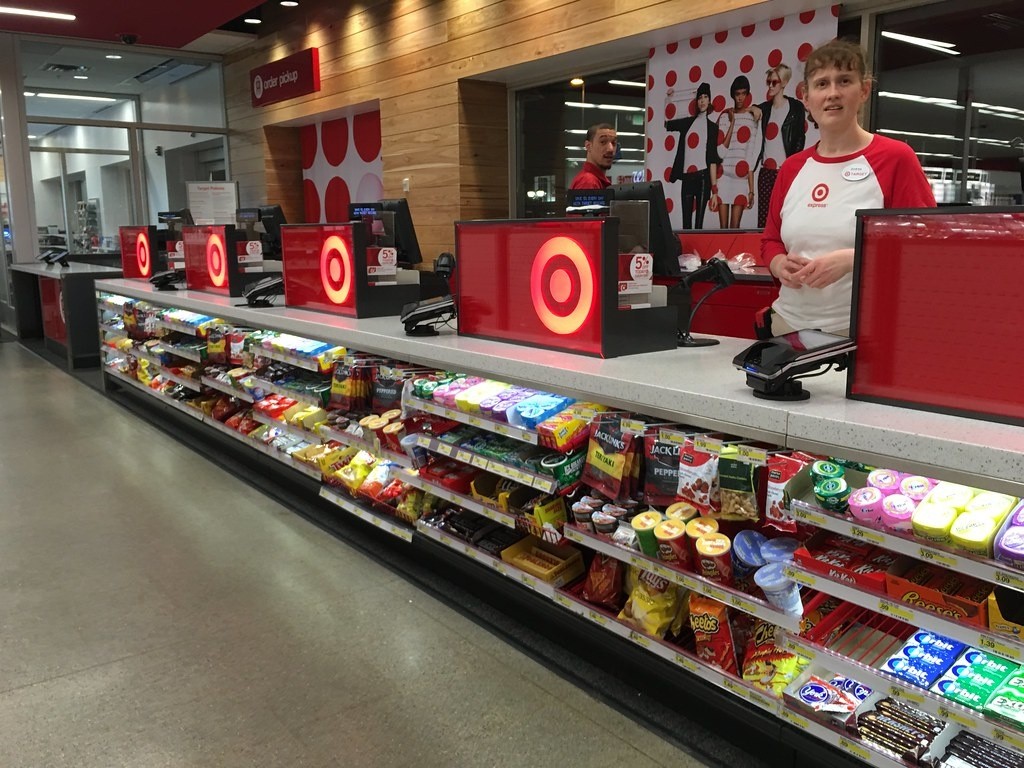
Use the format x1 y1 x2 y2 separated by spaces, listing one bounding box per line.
15 260 124 372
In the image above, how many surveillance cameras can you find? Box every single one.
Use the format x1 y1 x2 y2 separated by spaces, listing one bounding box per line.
121 34 137 44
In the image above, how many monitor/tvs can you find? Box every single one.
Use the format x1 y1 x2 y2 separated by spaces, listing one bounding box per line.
148 270 184 282
377 199 422 265
259 204 287 260
179 209 195 225
400 295 454 324
606 180 681 278
731 328 855 379
245 277 283 298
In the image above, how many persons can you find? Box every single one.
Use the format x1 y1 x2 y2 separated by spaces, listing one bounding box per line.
758 40 938 336
662 64 805 230
566 122 617 218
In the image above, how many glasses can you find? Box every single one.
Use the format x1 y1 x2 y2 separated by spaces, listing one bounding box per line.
766 79 781 88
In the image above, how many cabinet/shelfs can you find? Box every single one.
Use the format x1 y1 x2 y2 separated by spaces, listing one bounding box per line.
73 196 103 249
96 290 418 543
414 373 1021 768
